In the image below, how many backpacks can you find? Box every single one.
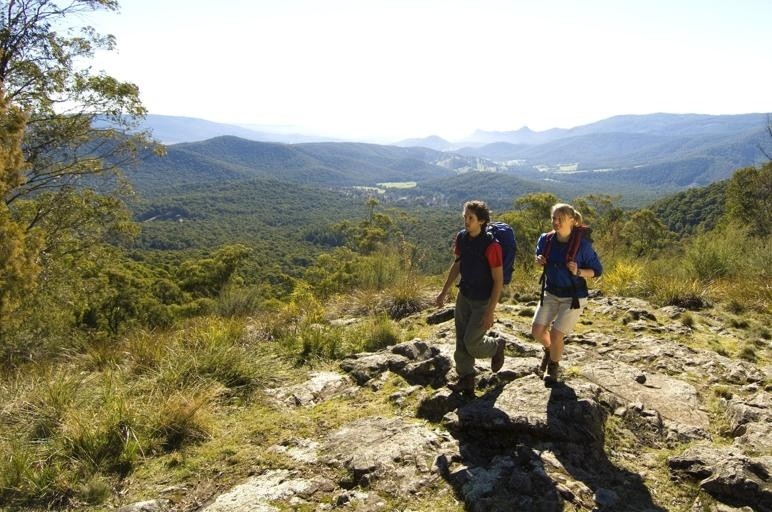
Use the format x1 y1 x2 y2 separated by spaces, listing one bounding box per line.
542 226 593 264
459 222 519 286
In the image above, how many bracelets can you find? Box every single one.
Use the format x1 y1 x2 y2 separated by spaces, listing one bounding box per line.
577 269 581 276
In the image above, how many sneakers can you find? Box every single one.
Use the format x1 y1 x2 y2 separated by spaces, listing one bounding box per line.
541 357 561 384
447 376 476 394
490 336 507 374
537 348 553 376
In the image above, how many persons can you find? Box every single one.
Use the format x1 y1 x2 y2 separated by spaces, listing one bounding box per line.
528 202 602 384
434 200 505 393
